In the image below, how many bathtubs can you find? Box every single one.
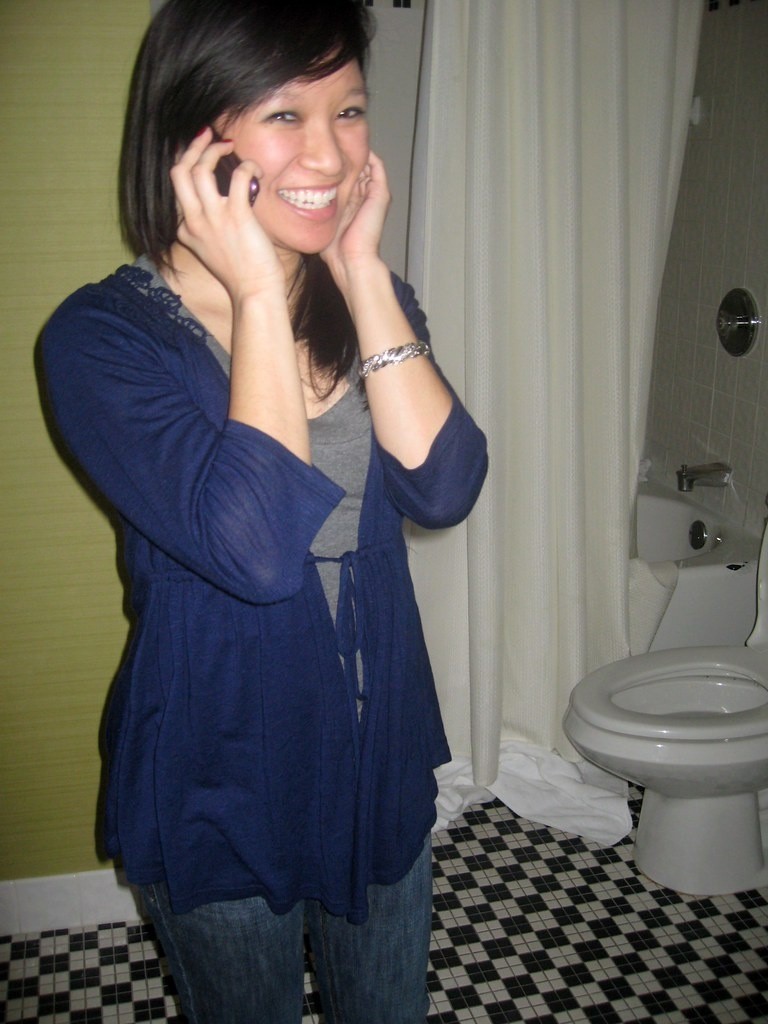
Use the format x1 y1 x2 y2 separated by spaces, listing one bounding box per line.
630 476 761 655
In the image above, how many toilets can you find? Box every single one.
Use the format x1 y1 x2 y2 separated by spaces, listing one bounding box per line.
562 520 768 895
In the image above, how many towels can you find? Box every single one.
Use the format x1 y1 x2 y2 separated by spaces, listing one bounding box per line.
431 739 633 847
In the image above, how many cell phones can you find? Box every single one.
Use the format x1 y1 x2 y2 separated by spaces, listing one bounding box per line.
207 129 260 209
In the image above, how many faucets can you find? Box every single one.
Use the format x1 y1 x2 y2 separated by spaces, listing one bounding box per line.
677 462 731 493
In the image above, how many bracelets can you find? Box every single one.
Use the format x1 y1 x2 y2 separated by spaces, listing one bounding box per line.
357 341 429 378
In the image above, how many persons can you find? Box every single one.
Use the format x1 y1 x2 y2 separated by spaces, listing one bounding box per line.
33 0 490 1023
